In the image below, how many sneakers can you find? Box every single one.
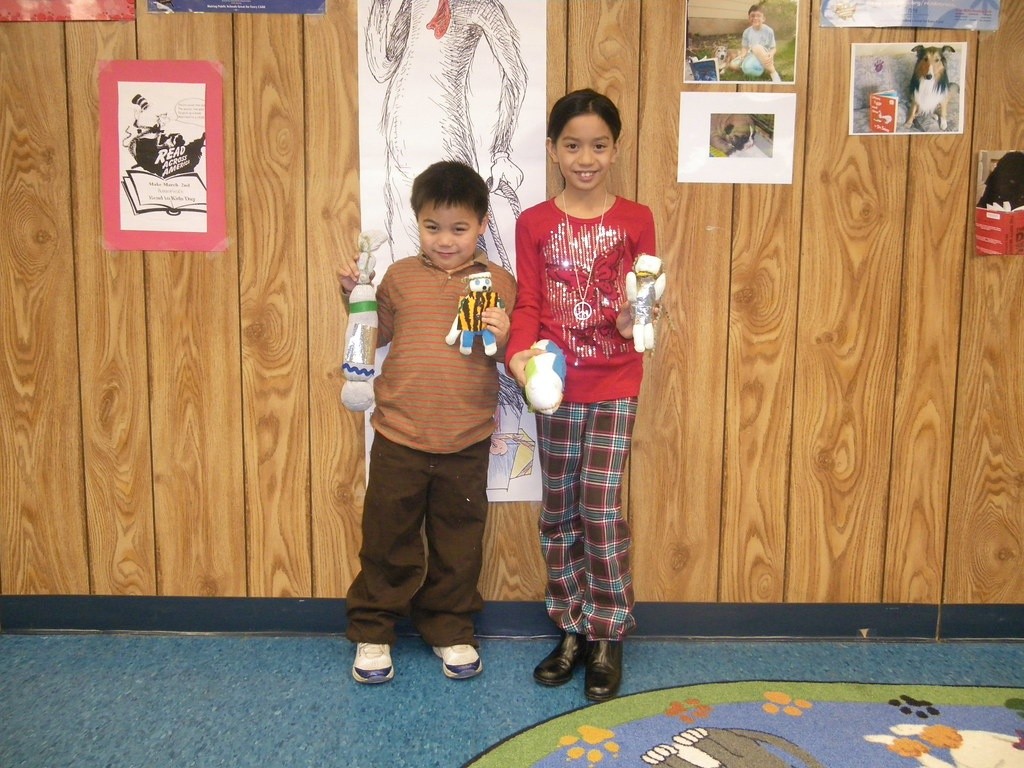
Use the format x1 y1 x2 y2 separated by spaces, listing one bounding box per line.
352 642 395 684
433 644 483 679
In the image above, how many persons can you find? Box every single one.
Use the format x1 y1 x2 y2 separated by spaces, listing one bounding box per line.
731 5 780 82
504 88 660 701
335 161 516 683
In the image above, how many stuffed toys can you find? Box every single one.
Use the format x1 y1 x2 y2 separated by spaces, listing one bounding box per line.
624 253 666 352
445 264 505 357
523 339 567 416
340 225 389 412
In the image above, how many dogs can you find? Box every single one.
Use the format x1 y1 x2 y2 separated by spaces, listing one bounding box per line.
710 113 757 156
712 42 743 74
904 44 960 131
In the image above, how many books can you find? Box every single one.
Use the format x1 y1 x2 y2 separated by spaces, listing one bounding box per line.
689 55 719 81
976 201 1024 256
869 90 899 132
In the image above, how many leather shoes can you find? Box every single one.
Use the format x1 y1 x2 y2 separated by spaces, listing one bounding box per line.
584 640 623 702
533 632 589 686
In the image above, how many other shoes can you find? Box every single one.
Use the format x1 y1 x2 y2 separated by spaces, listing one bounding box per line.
770 71 782 83
